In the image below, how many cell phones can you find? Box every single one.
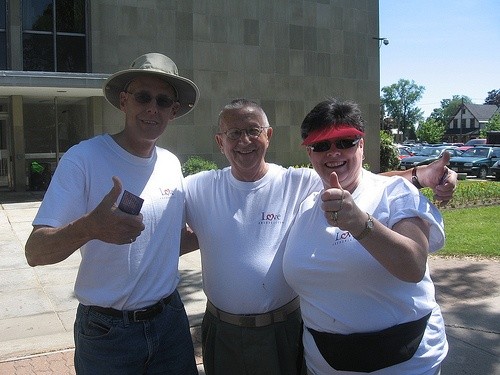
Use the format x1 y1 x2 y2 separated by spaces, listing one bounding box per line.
118 190 145 216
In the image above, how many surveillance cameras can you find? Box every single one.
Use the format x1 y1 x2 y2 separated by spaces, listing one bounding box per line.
384 39 389 45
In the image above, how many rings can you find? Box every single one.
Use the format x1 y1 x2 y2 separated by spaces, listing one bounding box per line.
131 238 134 244
331 212 338 221
334 220 337 226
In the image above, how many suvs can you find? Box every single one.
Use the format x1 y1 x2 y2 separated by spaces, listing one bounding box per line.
455 138 494 153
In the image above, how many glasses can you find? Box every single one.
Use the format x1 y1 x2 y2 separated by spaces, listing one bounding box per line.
121 90 177 107
217 127 270 140
308 139 360 152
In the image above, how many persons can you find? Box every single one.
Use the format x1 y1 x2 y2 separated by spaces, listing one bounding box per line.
25 52 199 375
183 99 458 375
283 97 449 375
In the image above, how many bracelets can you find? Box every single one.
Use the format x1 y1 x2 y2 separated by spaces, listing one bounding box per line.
412 167 424 189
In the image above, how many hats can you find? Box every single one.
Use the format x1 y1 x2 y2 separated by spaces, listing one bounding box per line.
103 53 200 121
301 125 365 146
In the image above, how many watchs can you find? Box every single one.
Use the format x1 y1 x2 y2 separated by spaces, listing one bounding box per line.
356 212 375 240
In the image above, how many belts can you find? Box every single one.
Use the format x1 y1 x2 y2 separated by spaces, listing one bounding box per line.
91 295 174 321
206 297 301 327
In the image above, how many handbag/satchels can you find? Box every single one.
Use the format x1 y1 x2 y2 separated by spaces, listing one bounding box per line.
305 311 432 373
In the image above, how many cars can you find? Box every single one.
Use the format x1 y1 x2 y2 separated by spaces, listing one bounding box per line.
404 142 464 147
447 146 500 181
395 146 463 170
